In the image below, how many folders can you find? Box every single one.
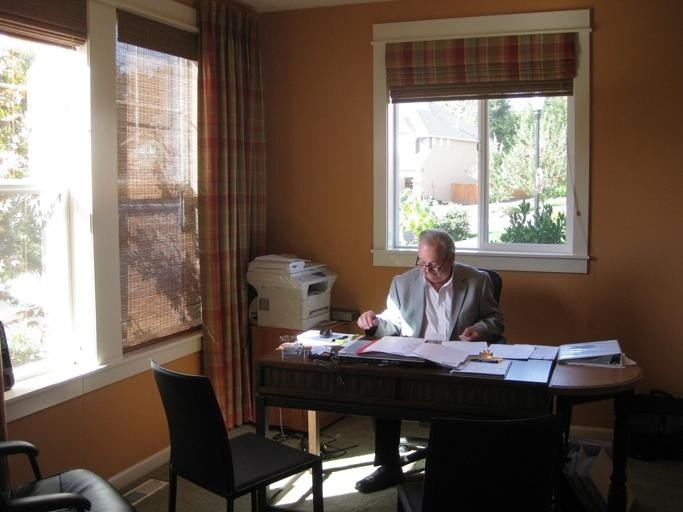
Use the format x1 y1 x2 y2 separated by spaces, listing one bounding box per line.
558 339 623 364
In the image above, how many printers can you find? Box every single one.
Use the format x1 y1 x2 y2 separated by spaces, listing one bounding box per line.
247 260 338 331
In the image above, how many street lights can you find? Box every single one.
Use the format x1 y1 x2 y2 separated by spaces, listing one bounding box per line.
531 97 544 217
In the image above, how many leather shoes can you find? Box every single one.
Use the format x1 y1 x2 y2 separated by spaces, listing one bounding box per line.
355 466 403 493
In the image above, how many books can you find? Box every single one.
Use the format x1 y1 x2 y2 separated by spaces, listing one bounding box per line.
336 334 622 378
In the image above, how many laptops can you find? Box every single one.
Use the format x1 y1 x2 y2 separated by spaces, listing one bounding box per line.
337 340 425 363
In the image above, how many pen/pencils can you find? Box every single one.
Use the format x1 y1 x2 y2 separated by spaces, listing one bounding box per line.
330 356 341 368
471 359 498 363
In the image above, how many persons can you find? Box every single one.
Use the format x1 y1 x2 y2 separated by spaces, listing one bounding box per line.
354 227 506 495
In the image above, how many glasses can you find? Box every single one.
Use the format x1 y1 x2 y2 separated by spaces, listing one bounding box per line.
415 251 449 271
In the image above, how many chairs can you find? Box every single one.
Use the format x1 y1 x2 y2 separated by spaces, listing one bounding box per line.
146 356 324 512
396 411 576 511
0 437 135 512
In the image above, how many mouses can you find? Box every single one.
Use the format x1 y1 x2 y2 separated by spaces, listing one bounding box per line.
320 329 333 338
